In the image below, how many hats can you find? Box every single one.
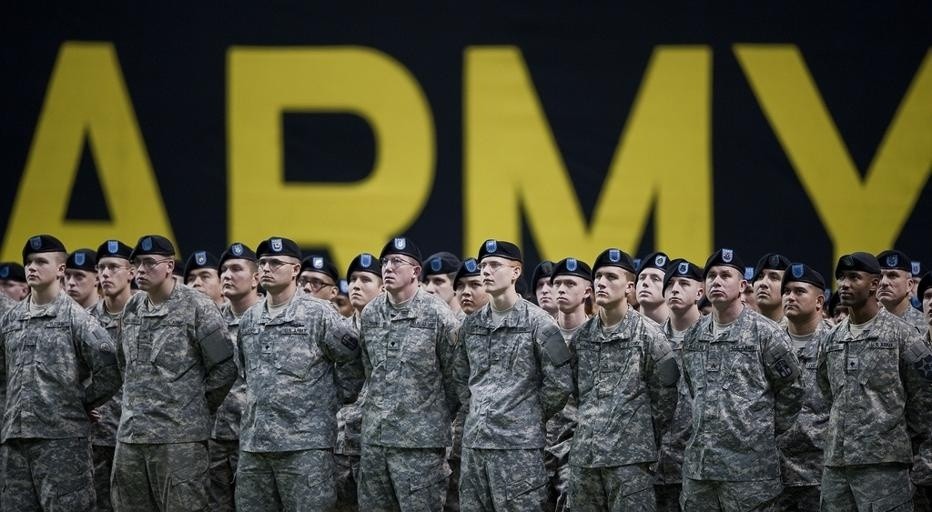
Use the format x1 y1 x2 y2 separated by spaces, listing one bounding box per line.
183 237 338 282
1 235 176 281
875 249 913 273
835 251 881 279
346 237 522 289
531 248 824 291
911 262 922 278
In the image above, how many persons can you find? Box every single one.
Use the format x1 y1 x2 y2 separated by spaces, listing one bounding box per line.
1 233 932 512
